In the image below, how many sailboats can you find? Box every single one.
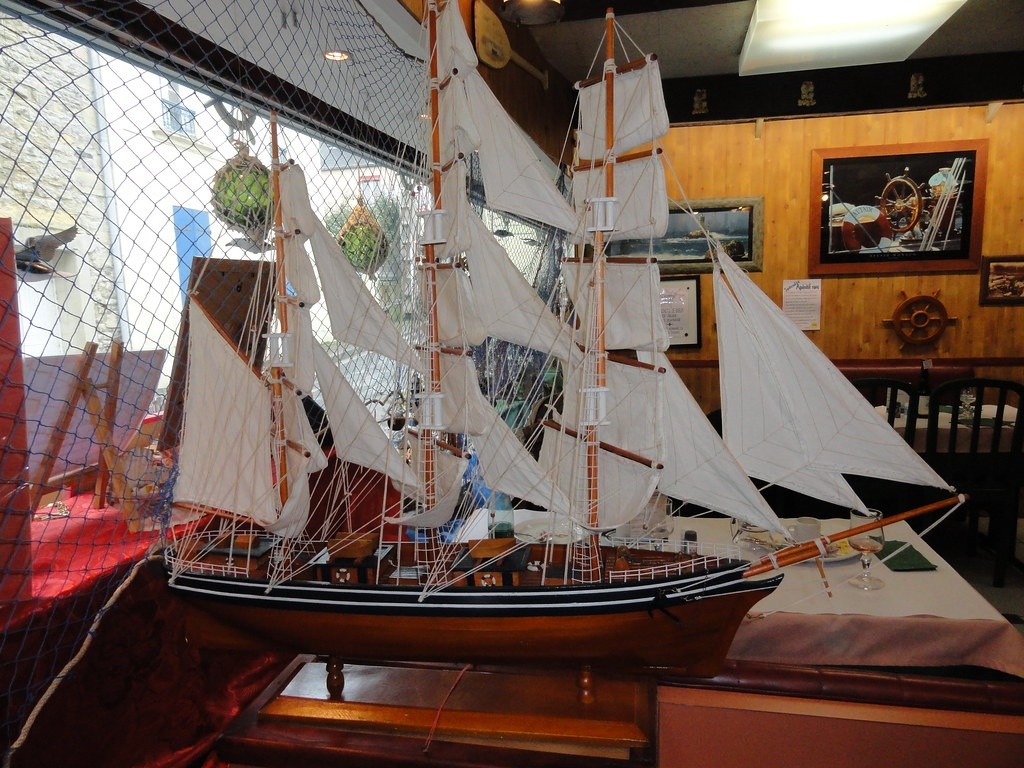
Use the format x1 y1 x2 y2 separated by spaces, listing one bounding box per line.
163 5 1002 680
